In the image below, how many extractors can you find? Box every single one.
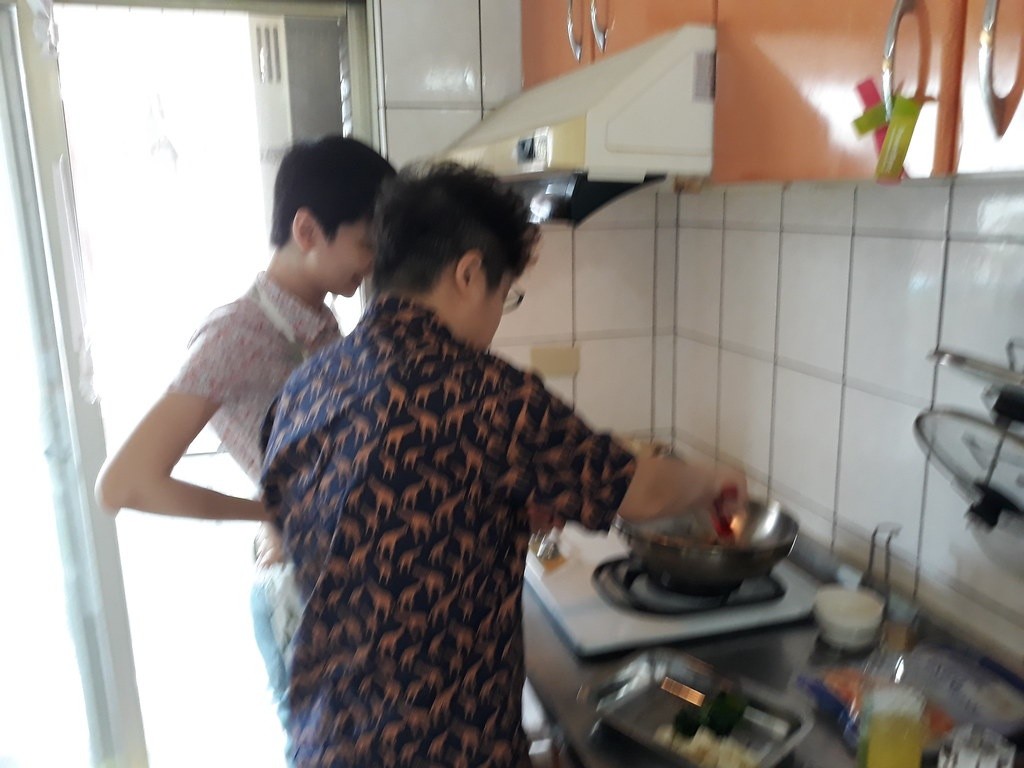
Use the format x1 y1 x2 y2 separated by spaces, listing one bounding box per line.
439 25 715 228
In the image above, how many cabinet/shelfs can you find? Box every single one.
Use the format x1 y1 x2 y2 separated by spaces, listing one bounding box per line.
519 1 715 91
710 0 1024 183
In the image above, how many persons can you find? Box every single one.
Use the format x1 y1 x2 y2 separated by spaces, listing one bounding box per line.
93 132 397 767
256 156 748 768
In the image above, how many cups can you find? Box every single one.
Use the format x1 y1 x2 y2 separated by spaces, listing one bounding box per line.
867 683 926 768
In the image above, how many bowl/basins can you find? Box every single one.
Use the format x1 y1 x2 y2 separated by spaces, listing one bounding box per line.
817 588 883 649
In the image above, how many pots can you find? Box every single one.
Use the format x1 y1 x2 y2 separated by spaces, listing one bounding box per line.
622 499 797 588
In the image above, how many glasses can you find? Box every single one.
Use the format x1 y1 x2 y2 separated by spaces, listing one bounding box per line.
446 249 525 314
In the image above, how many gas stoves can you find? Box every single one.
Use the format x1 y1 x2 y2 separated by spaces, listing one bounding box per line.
524 519 814 656
